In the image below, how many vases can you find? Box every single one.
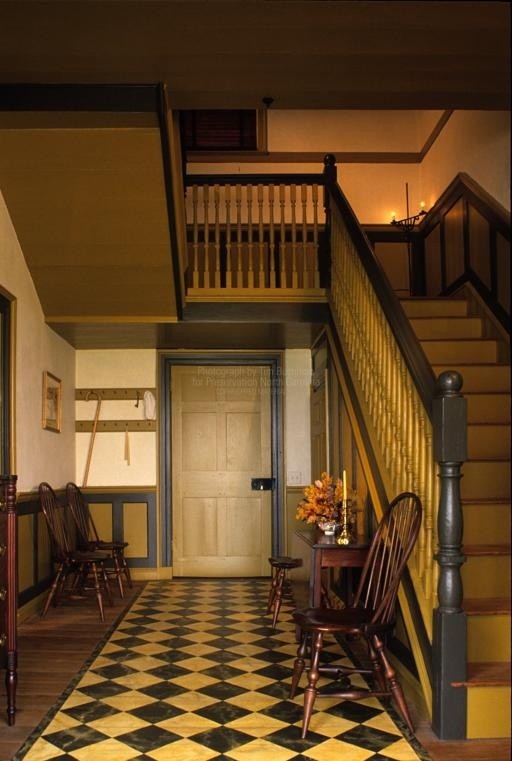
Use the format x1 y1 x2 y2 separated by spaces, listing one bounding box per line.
316 521 340 535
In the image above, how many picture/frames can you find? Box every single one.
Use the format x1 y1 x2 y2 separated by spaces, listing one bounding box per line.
41 369 63 433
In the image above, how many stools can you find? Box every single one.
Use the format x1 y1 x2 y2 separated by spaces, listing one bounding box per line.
265 555 308 629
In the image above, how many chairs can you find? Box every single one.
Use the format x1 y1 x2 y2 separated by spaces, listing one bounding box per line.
287 486 428 739
66 483 133 599
39 482 125 623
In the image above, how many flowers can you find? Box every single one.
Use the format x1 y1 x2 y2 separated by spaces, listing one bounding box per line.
294 471 366 525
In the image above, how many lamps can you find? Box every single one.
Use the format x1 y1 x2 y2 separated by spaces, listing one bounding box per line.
391 199 430 234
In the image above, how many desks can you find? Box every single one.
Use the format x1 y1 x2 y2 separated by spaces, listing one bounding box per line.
294 527 382 619
